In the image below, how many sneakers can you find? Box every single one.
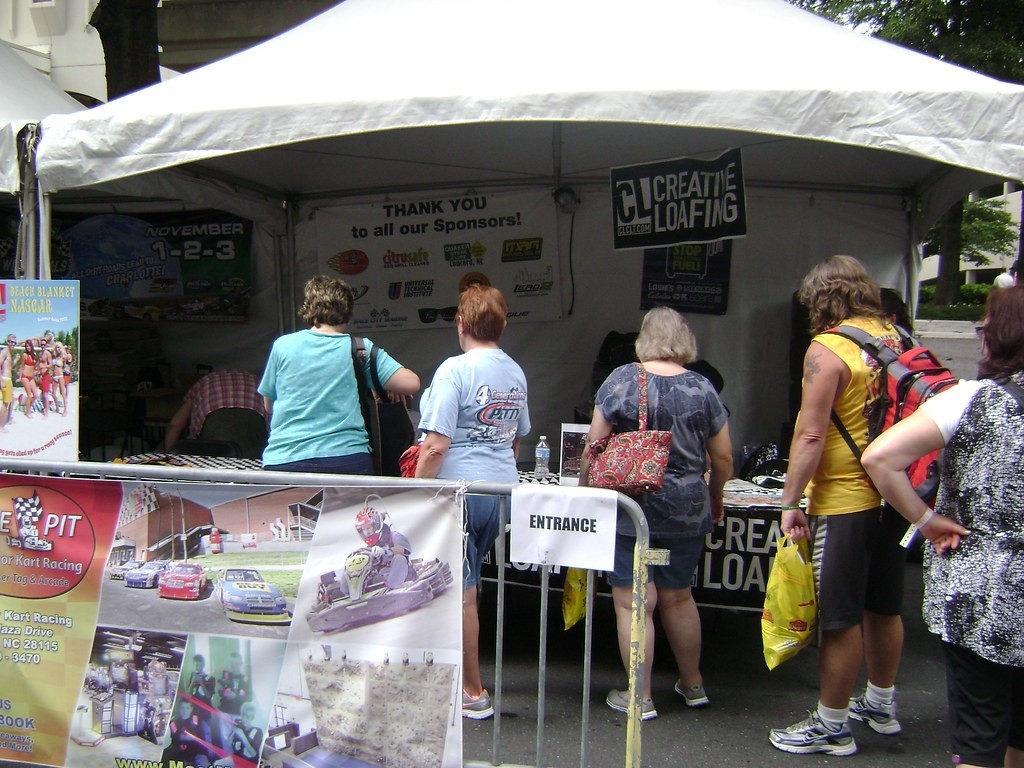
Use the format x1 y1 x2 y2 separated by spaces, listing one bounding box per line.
462 688 495 720
848 688 901 734
676 680 709 706
607 689 657 721
769 709 857 756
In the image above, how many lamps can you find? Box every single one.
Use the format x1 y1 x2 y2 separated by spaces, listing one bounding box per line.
553 185 579 216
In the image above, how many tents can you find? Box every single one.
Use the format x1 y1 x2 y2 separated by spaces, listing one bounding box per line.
0 0 1024 410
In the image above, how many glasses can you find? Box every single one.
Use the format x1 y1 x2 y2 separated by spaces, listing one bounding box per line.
41 343 46 347
10 341 17 344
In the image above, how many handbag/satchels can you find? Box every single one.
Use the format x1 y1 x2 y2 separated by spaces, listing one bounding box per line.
588 362 672 491
352 335 416 477
563 567 586 629
762 533 817 668
398 446 419 477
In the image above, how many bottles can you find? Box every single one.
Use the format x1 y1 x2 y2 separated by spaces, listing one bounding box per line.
211 527 221 553
533 435 550 481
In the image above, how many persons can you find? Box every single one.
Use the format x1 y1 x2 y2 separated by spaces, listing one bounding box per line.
337 507 418 592
0 330 73 433
88 331 127 405
768 254 914 755
172 652 263 768
579 308 734 721
977 272 1014 379
859 287 1024 768
165 368 269 458
260 275 421 477
416 283 531 720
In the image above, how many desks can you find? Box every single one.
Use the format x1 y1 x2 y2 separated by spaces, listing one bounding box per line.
124 454 821 652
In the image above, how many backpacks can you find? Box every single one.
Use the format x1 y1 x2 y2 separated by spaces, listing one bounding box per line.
821 318 960 509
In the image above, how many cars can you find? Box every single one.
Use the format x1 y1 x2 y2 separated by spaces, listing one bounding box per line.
125 560 168 588
215 567 292 624
109 560 147 580
158 563 207 599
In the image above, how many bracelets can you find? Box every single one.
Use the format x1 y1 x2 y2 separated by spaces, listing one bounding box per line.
899 508 934 548
780 504 799 509
